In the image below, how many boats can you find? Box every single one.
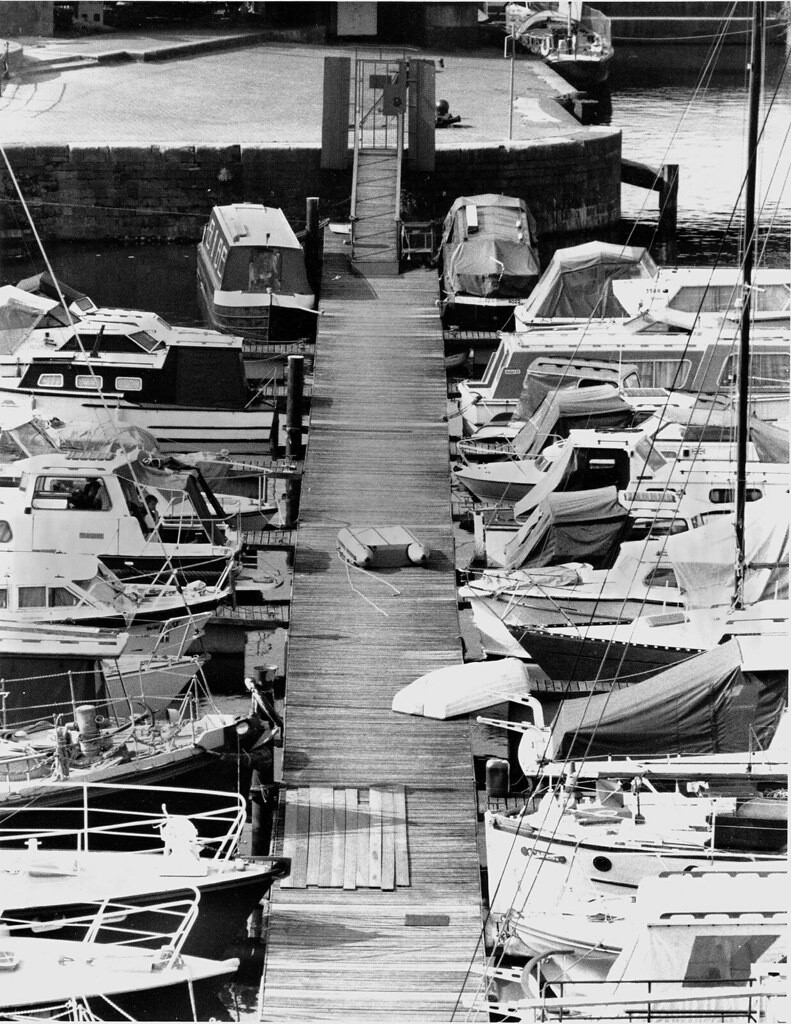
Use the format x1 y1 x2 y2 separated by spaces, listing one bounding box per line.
498 1 622 86
2 184 789 1024
194 200 317 337
